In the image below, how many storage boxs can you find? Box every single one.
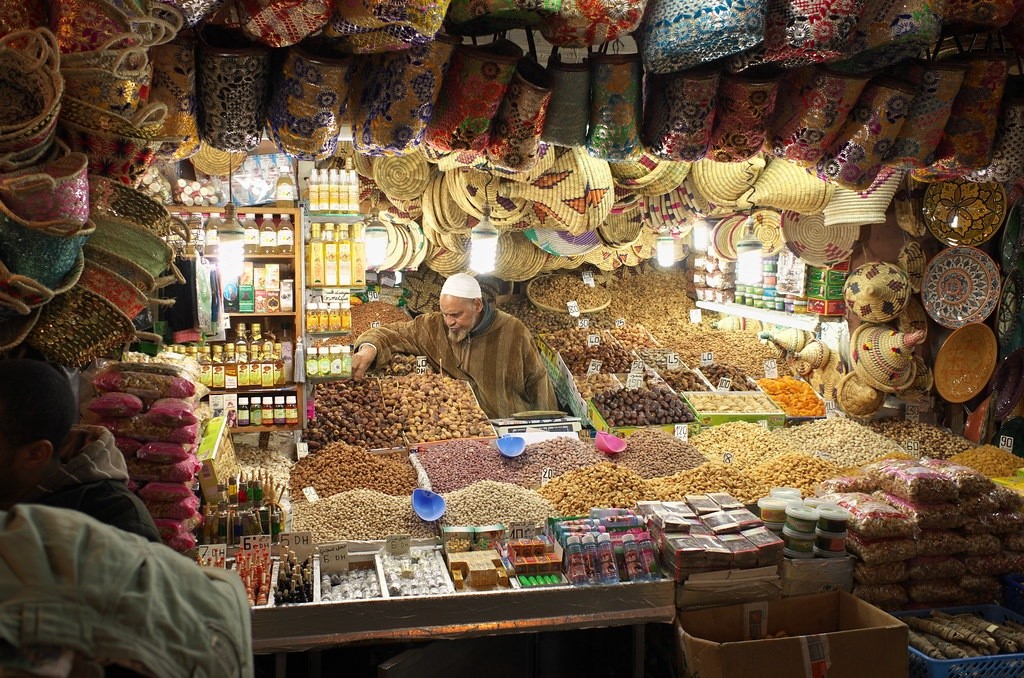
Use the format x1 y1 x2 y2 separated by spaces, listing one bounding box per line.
675 573 1024 678
808 266 849 316
196 416 237 503
539 326 827 436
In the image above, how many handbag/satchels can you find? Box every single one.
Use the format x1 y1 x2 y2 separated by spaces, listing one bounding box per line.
150 0 1024 190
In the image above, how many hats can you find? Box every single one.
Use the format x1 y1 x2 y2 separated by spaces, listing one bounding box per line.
711 260 926 418
499 147 589 237
691 157 833 216
441 273 482 298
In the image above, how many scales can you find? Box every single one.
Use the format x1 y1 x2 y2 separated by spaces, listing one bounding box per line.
488 410 582 438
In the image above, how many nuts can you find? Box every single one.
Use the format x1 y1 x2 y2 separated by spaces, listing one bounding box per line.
235 417 908 546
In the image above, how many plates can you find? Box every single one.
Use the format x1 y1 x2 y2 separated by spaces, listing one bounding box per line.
922 171 1007 249
934 321 997 404
921 245 1001 327
964 187 1024 456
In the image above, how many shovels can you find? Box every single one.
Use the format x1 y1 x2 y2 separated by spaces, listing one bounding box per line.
594 431 627 454
408 453 446 522
495 433 527 458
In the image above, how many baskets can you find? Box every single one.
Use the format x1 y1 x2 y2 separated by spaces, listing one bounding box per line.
572 146 614 232
423 171 471 254
888 574 1024 678
822 166 903 226
0 0 184 370
895 169 933 401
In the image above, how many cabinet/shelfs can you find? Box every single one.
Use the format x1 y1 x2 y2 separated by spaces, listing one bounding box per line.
152 206 308 452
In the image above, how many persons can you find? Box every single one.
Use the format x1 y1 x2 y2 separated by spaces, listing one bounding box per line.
0 361 165 545
350 273 560 420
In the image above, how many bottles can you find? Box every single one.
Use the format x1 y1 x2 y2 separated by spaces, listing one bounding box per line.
304 167 367 380
198 476 312 604
152 318 295 389
164 212 297 259
273 168 294 208
236 396 300 427
